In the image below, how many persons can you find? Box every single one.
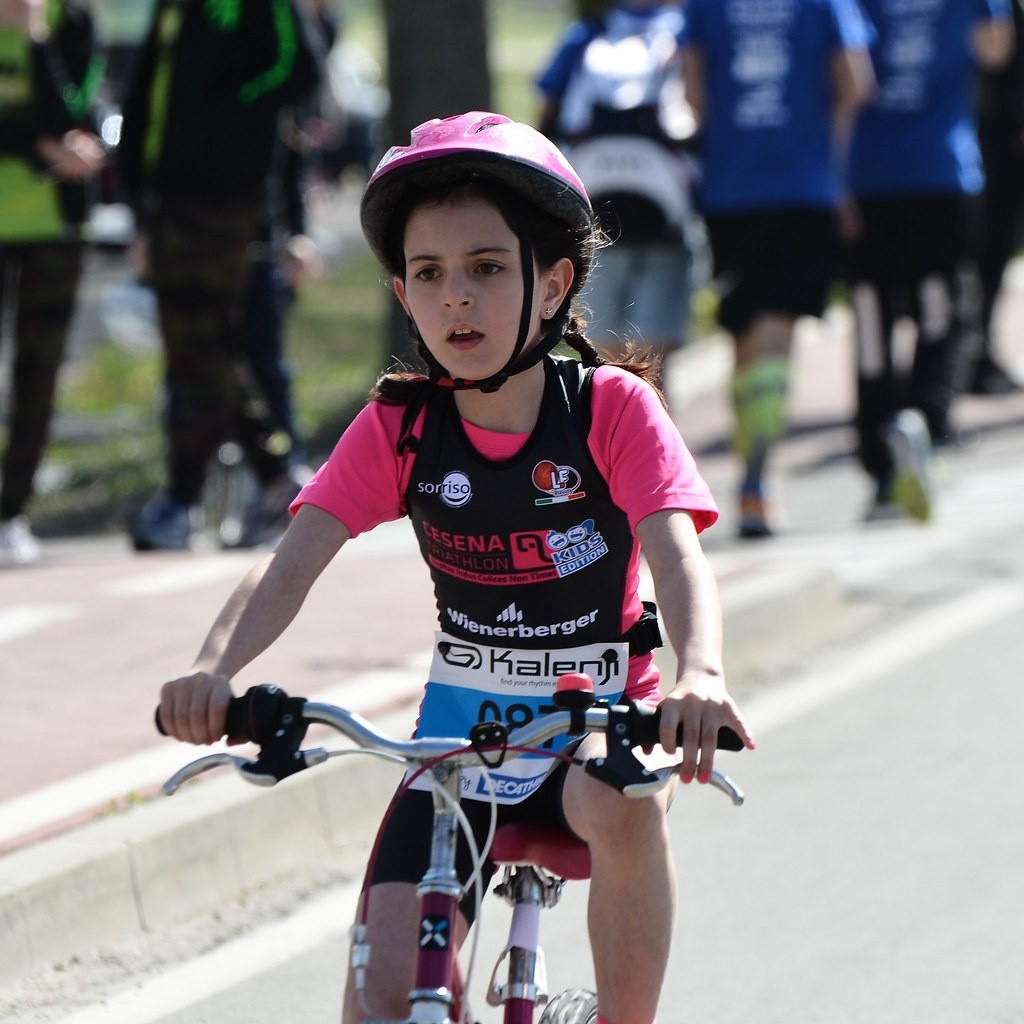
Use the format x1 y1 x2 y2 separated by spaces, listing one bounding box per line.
158 112 757 1023
535 0 705 407
841 1 1011 518
0 0 337 555
954 1 1024 395
673 0 876 536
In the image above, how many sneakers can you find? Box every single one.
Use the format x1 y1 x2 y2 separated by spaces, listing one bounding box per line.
2 350 1023 563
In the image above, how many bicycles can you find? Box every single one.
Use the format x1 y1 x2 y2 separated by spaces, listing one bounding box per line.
152 682 746 1024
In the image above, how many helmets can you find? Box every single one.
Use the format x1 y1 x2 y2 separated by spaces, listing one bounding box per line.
361 111 597 390
